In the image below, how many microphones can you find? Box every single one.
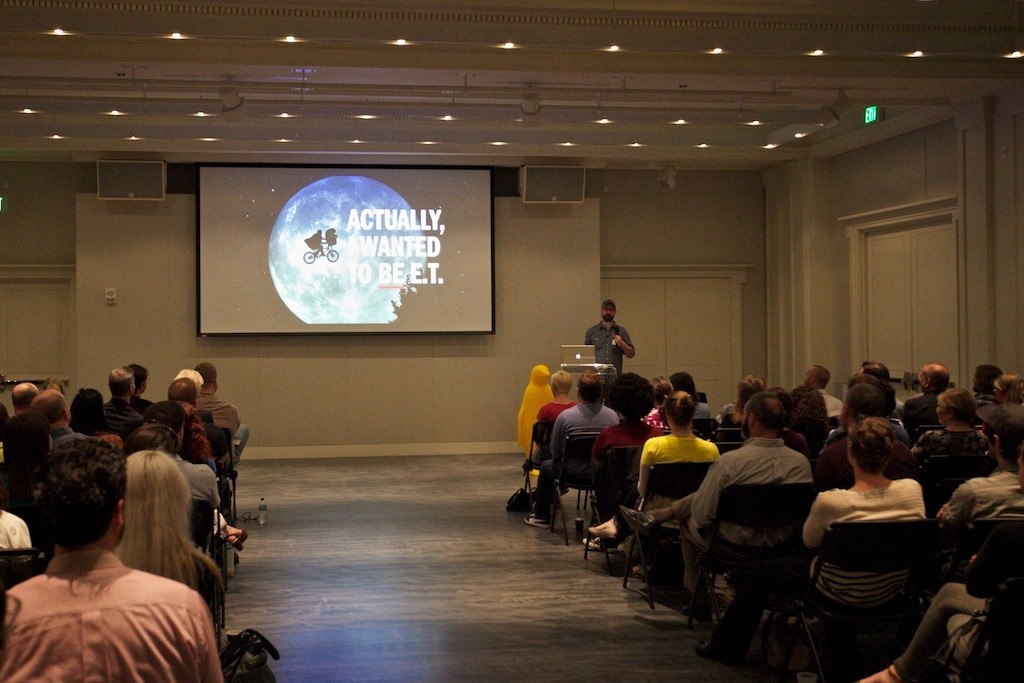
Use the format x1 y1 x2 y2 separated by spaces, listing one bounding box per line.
613 326 618 348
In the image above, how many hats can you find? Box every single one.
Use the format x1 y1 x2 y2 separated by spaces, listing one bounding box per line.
975 402 1024 439
602 299 616 309
174 368 204 390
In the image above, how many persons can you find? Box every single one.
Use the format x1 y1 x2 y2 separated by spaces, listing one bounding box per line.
0 361 250 683
585 299 635 375
523 361 1024 683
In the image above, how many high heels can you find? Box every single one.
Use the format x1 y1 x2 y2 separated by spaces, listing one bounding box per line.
227 527 248 551
234 553 239 565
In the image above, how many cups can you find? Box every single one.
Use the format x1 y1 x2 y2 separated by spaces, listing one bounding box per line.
576 519 583 531
796 673 817 683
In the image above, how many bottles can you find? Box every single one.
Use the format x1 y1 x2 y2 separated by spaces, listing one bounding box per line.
257 497 267 524
225 542 234 578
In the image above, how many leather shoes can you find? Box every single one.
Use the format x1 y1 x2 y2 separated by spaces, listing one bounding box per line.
619 503 661 536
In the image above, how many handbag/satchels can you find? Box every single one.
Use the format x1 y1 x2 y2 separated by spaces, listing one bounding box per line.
220 629 280 683
505 487 537 512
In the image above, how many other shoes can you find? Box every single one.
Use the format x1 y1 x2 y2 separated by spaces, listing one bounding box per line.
633 563 668 580
582 538 602 550
661 589 692 605
723 570 734 582
695 638 746 667
524 513 551 529
557 485 570 496
680 598 713 623
618 537 639 552
588 526 618 539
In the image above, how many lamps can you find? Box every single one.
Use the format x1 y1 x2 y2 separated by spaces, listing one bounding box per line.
520 86 541 116
654 165 678 192
815 99 849 130
218 88 246 114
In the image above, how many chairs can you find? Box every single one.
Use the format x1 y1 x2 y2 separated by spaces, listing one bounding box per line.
523 418 1024 683
0 410 252 647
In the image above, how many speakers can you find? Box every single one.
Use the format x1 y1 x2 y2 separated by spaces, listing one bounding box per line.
95 158 165 202
518 166 585 204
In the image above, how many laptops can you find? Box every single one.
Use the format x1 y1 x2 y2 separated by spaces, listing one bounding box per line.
560 344 600 365
